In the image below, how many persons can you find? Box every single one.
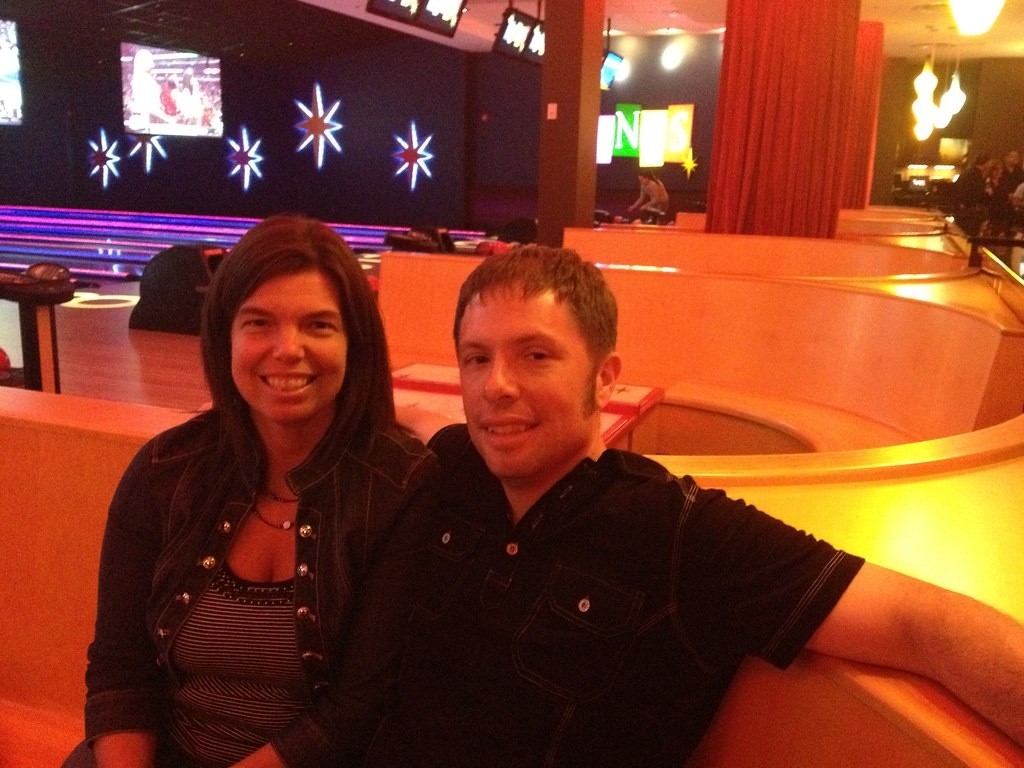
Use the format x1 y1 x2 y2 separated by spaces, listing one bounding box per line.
628 170 669 220
357 245 1024 768
131 50 221 126
58 214 438 768
951 151 1024 238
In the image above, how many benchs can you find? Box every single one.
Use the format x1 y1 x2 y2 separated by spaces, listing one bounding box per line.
1 205 1024 768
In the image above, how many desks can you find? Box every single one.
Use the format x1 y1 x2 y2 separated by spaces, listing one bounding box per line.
388 364 666 461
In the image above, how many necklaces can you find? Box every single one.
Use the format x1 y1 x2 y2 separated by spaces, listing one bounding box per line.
251 492 298 529
